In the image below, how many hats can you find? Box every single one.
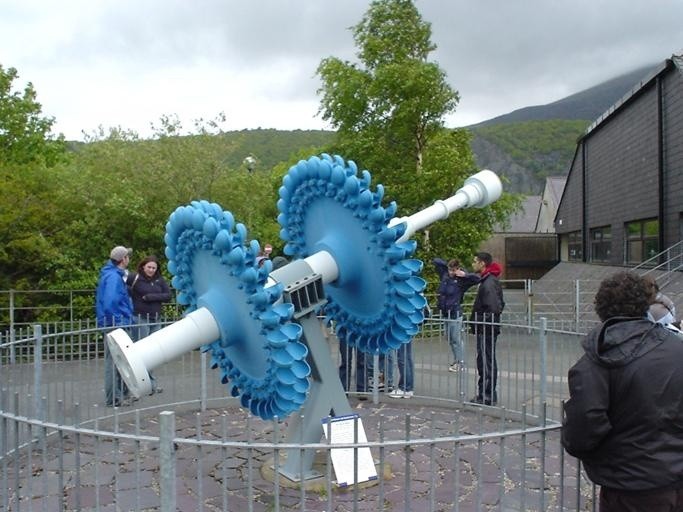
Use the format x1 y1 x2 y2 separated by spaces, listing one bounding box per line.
110 245 133 261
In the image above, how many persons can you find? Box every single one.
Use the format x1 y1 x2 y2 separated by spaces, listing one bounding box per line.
126 257 172 395
256 244 289 269
339 339 413 400
433 257 480 372
96 246 133 406
469 252 505 406
468 404 469 406
562 272 683 512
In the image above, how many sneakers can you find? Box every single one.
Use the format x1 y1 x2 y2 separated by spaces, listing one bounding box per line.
366 381 414 399
106 397 134 408
449 362 465 373
468 397 499 407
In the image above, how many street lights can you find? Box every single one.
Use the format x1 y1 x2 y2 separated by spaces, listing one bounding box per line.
241 155 255 174
